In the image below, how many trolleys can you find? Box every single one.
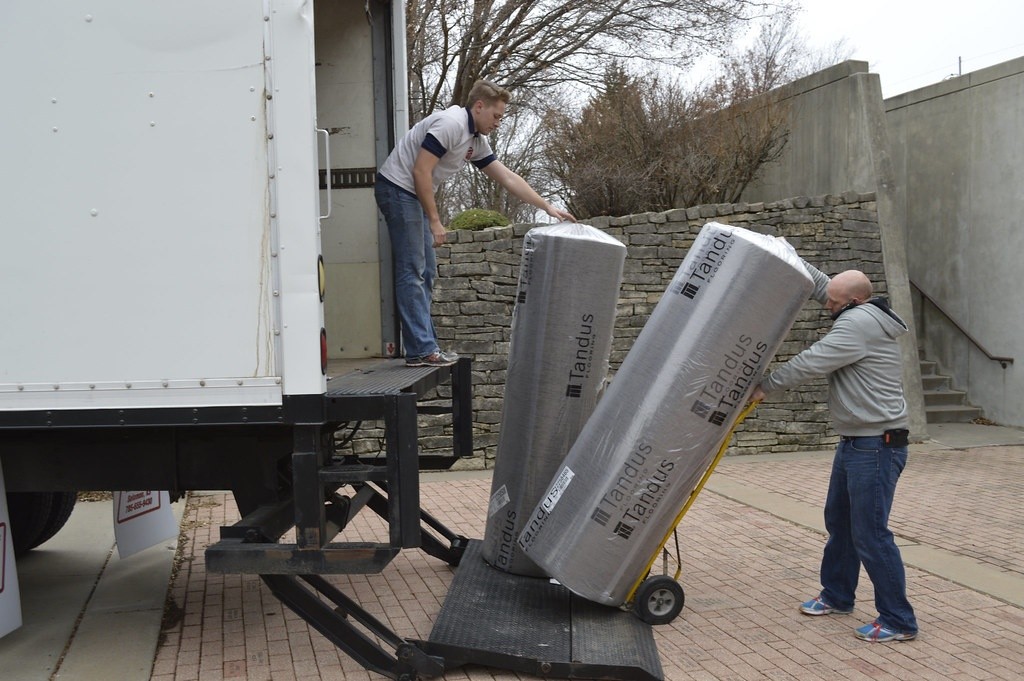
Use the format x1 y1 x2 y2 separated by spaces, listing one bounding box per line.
618 399 760 625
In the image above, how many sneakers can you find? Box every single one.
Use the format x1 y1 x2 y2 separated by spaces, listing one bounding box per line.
406 351 459 367
854 621 916 642
798 596 851 615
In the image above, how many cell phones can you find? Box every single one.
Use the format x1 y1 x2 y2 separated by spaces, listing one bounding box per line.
831 302 855 321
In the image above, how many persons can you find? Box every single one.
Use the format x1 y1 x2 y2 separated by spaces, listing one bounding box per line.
374 81 578 367
750 258 919 642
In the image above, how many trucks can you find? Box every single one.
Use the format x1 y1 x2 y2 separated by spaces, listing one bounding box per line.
1 0 668 680
